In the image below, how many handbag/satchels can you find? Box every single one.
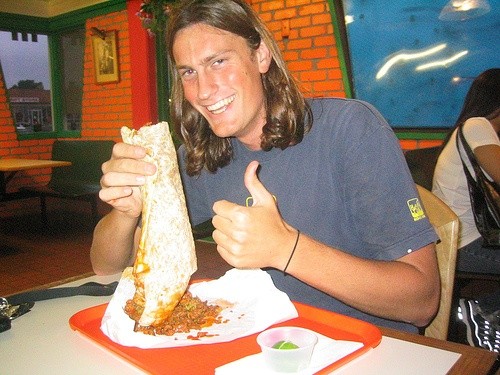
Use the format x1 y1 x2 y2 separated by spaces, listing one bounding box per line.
467 171 500 249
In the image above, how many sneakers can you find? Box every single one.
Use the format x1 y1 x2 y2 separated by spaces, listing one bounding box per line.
459 297 500 361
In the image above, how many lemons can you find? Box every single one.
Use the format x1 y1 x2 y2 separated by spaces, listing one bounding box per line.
279 342 300 350
272 341 285 350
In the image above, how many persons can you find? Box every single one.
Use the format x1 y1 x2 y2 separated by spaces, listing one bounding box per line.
88 0 441 335
433 70 500 354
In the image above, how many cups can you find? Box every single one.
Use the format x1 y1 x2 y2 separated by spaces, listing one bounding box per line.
255 325 319 373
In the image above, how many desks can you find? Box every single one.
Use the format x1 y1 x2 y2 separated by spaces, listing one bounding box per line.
0 158 72 202
0 271 498 375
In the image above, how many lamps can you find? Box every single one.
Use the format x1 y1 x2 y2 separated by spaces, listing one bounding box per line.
90 27 105 40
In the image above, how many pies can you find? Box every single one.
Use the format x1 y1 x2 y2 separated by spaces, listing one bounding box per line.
120 121 198 326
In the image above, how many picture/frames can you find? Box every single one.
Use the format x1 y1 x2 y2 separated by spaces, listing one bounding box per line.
91 29 120 86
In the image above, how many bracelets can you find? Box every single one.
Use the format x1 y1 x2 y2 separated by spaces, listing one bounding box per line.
282 227 300 275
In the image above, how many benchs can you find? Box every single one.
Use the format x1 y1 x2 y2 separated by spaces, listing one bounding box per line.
19 140 116 228
